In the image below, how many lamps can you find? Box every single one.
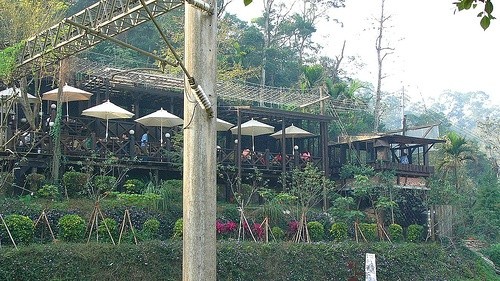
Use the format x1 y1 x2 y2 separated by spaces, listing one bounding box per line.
234 138 239 144
165 133 170 138
51 103 57 110
129 130 135 135
294 145 299 151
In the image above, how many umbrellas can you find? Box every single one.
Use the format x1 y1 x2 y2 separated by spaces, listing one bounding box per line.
0 85 41 135
230 117 275 152
80 99 135 142
42 83 93 121
270 123 313 154
133 107 183 161
217 117 234 131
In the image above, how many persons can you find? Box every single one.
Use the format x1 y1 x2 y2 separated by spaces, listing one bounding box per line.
275 152 282 161
141 128 150 148
400 153 409 164
121 133 129 140
243 147 251 157
302 151 312 161
